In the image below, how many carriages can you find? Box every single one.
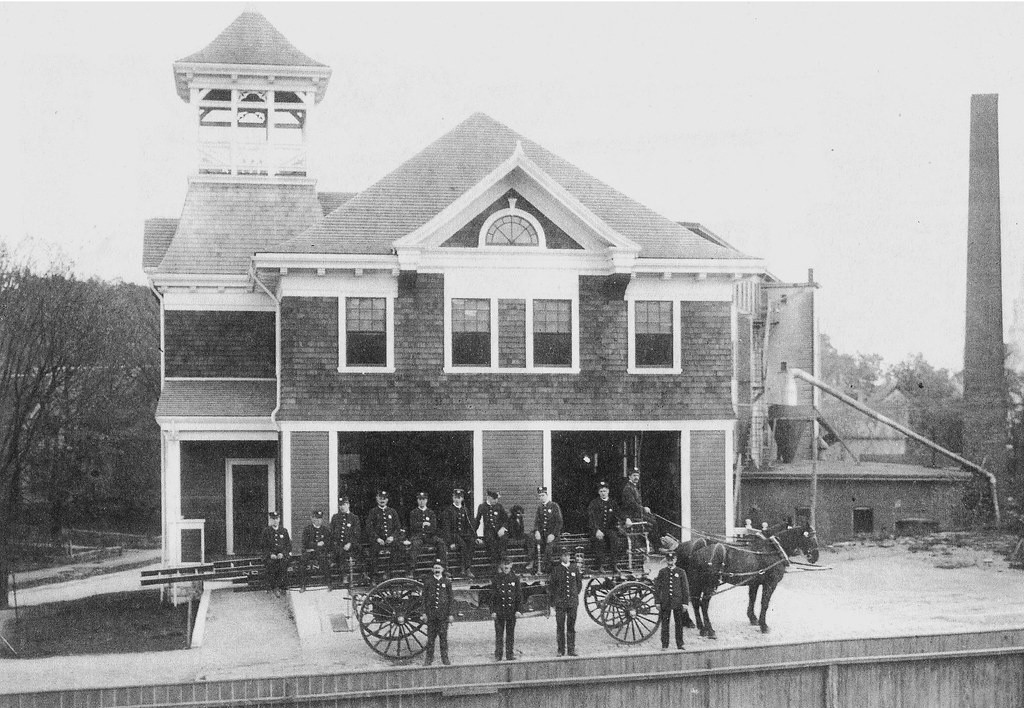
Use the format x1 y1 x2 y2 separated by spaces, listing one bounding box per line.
584 515 819 644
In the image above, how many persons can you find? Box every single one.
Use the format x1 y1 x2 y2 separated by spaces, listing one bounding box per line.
490 556 523 661
547 545 583 657
263 467 673 597
653 551 690 651
419 556 455 666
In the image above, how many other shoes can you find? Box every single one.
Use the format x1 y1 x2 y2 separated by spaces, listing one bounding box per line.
298 584 308 593
556 650 565 656
567 650 578 656
506 656 517 660
459 567 475 578
422 658 434 665
526 560 534 570
441 657 451 664
494 656 502 661
661 646 668 650
676 644 686 649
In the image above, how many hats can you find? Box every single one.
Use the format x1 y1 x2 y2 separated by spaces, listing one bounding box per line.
502 558 513 564
378 490 390 498
487 489 500 499
666 551 677 558
431 559 445 567
628 467 640 473
598 482 609 489
338 498 349 506
536 486 548 493
416 493 429 499
314 511 323 518
559 548 571 554
269 511 280 517
453 489 464 496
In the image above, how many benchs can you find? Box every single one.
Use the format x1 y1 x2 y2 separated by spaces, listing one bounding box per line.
233 523 652 591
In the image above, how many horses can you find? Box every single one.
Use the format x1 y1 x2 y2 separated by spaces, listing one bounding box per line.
674 522 788 629
686 522 819 640
